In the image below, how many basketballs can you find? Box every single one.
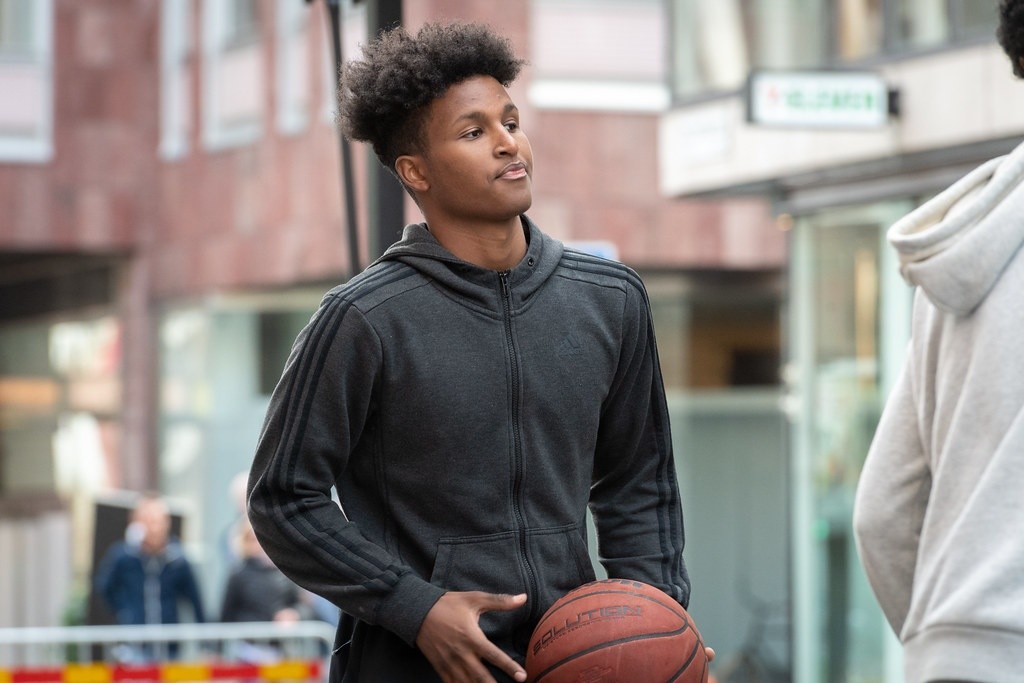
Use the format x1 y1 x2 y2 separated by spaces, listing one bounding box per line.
523 577 710 683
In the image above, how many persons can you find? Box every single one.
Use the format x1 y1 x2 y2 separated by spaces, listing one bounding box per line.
93 497 337 663
854 0 1024 683
246 26 717 683
